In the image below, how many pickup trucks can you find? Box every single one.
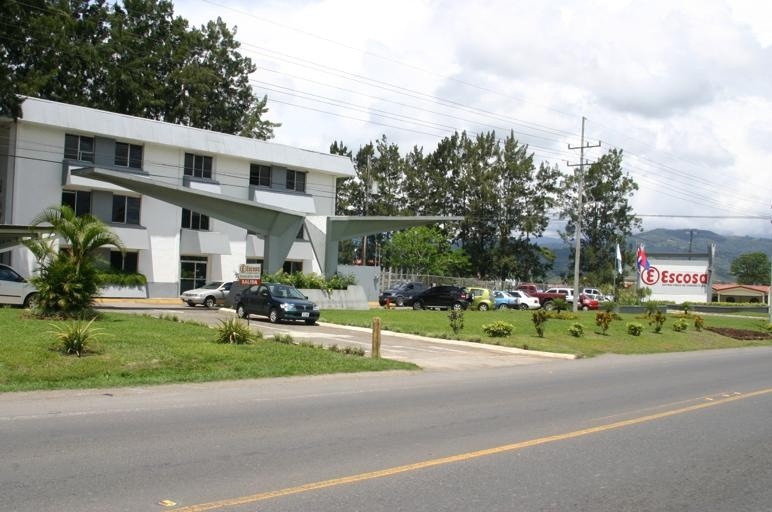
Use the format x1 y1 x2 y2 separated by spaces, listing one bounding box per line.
515 285 567 311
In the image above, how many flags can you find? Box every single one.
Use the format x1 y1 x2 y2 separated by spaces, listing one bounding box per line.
616 244 623 275
636 244 650 273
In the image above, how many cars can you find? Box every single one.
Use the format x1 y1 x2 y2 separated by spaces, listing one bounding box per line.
378 282 427 307
577 287 610 310
0 263 42 309
182 280 235 306
441 286 495 311
544 288 580 311
237 284 320 323
407 286 471 311
493 289 540 310
